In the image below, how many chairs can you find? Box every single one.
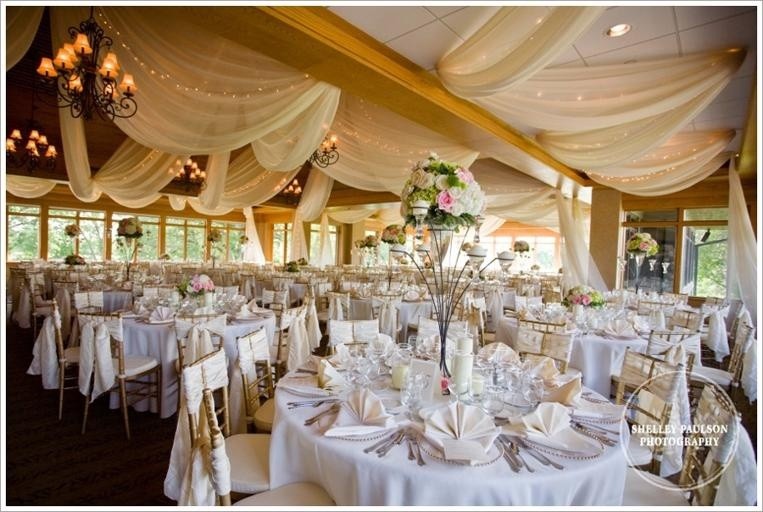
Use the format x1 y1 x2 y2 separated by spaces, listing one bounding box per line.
353 319 379 343
326 320 352 349
236 325 275 433
483 283 502 322
616 347 685 476
16 278 54 342
173 312 230 379
271 276 300 306
518 278 527 295
326 292 351 320
515 296 526 312
74 308 161 440
289 283 308 308
26 298 79 420
303 285 322 350
239 274 265 305
689 322 753 400
645 330 701 364
282 297 322 354
700 297 726 307
164 366 338 506
180 345 270 494
539 279 563 290
159 284 176 298
515 325 574 374
516 317 568 333
270 302 288 384
416 315 468 337
69 288 103 347
528 296 543 304
618 383 741 506
466 296 496 343
493 287 517 332
287 318 324 370
470 303 485 349
667 308 705 343
545 290 563 304
699 299 745 369
141 285 159 296
8 267 30 319
625 388 675 475
259 288 287 320
371 305 402 343
662 290 689 307
635 299 676 317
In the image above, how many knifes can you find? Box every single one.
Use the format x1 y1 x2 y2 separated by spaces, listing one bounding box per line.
364 428 404 455
495 442 520 473
499 437 522 467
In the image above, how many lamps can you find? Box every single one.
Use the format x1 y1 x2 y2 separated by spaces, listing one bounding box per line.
6 105 58 163
312 127 340 167
271 173 301 197
606 23 632 38
37 6 137 122
166 155 211 191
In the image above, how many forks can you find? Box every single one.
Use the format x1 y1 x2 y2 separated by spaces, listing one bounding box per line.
288 401 325 408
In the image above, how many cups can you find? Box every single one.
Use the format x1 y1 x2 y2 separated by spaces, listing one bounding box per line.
452 350 474 396
468 377 484 406
401 371 424 424
506 368 522 402
523 376 543 405
482 382 504 426
456 334 473 355
397 343 411 358
407 336 420 352
392 356 410 390
356 358 370 383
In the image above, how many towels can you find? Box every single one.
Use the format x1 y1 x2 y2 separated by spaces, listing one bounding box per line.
423 333 454 352
404 290 419 301
247 298 271 312
324 387 397 436
477 343 518 362
149 305 175 323
522 353 559 380
275 359 348 396
509 402 603 455
139 295 150 304
650 308 665 330
121 300 148 317
236 305 255 319
213 291 225 304
540 372 606 408
600 319 637 338
192 305 214 321
626 311 649 334
356 288 370 300
231 294 246 305
519 309 535 320
561 435 566 438
370 334 391 355
310 343 354 370
409 401 502 465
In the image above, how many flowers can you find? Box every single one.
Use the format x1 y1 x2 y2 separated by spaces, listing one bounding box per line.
206 229 223 242
64 225 81 236
355 239 365 249
65 255 86 266
381 225 406 247
284 260 297 272
117 218 143 237
626 232 659 257
400 153 487 229
511 239 534 253
366 234 379 250
184 275 215 293
560 285 608 309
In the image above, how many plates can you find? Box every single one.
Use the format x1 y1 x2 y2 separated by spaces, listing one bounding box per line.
144 319 174 324
520 427 605 460
573 398 621 424
237 316 262 323
416 439 505 468
122 314 142 320
331 428 393 441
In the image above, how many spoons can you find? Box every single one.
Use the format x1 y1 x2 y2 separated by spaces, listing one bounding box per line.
509 442 535 473
